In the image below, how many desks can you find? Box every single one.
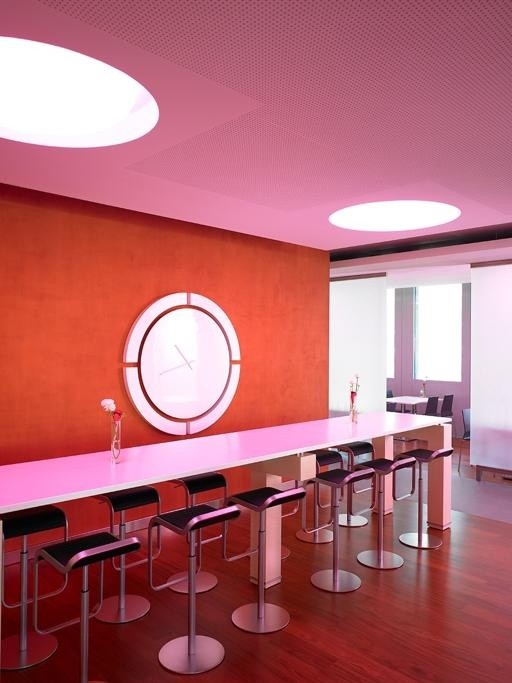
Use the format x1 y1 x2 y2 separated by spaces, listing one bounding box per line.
387 395 444 414
0 411 453 588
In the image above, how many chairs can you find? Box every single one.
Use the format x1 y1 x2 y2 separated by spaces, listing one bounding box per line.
387 389 396 412
458 409 470 472
421 397 438 416
441 395 454 417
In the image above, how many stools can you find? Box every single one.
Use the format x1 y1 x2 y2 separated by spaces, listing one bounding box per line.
294 442 456 593
0 470 307 676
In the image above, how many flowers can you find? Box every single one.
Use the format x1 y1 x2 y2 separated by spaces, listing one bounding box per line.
347 374 359 411
421 382 426 393
101 399 124 441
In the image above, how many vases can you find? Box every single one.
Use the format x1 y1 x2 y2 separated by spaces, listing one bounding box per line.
351 403 358 423
110 421 123 464
422 388 426 397
